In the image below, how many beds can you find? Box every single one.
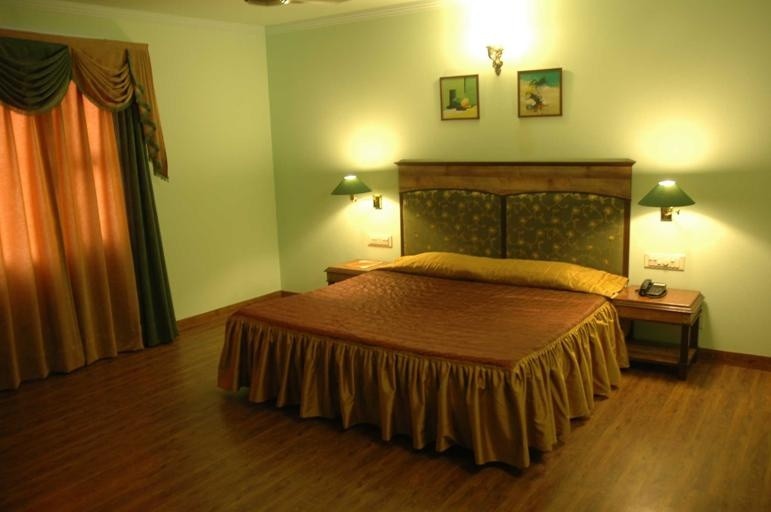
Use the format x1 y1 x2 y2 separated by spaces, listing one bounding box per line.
224 158 634 465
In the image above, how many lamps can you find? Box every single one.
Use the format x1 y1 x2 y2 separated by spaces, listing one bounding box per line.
331 175 382 210
636 181 695 223
483 31 506 75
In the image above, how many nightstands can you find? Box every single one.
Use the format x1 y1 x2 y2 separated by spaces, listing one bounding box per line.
612 285 704 381
324 260 387 285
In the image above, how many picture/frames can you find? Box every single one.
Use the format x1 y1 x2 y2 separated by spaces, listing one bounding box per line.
439 74 480 121
516 67 562 119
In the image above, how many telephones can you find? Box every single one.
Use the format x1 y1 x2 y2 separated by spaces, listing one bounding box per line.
639 279 667 298
